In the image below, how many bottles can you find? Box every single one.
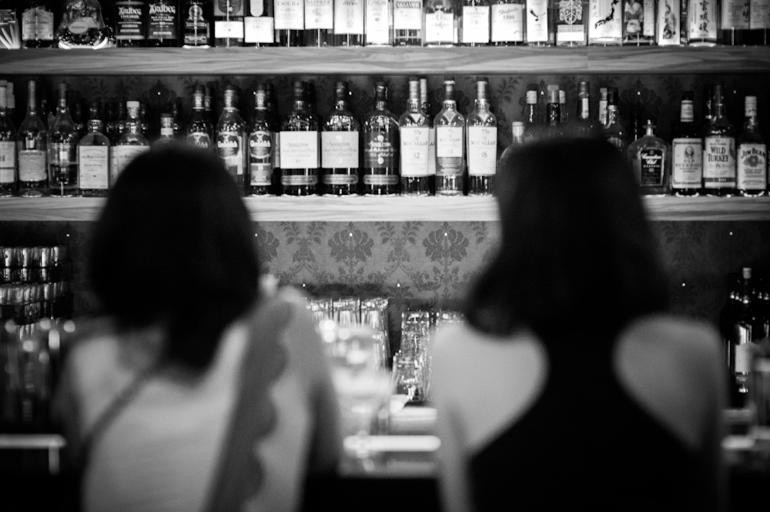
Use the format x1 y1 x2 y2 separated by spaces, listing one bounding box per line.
526 84 538 126
49 81 76 196
469 77 499 194
22 0 53 47
627 119 667 187
459 0 490 46
689 0 718 48
183 81 212 146
244 0 275 47
510 121 525 141
555 0 587 46
278 82 317 196
215 85 244 193
577 81 590 118
728 265 770 408
750 0 770 46
545 84 561 127
738 86 766 196
491 0 523 47
214 0 243 48
0 245 67 323
362 81 398 194
558 89 567 123
424 0 458 48
657 0 689 47
321 83 359 194
111 100 175 184
720 0 750 46
115 0 147 48
275 0 304 47
79 119 109 195
394 0 423 47
703 88 736 199
364 0 394 47
180 1 214 48
248 84 274 195
305 0 333 47
604 105 625 148
17 80 46 197
524 0 554 46
598 87 608 125
149 1 179 47
0 84 16 198
401 78 432 196
623 0 656 46
334 1 364 47
434 78 466 194
588 0 622 46
669 89 702 195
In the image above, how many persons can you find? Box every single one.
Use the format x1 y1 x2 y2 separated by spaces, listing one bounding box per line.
429 135 725 511
51 142 343 511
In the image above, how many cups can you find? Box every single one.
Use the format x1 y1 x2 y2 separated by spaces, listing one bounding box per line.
392 309 463 404
